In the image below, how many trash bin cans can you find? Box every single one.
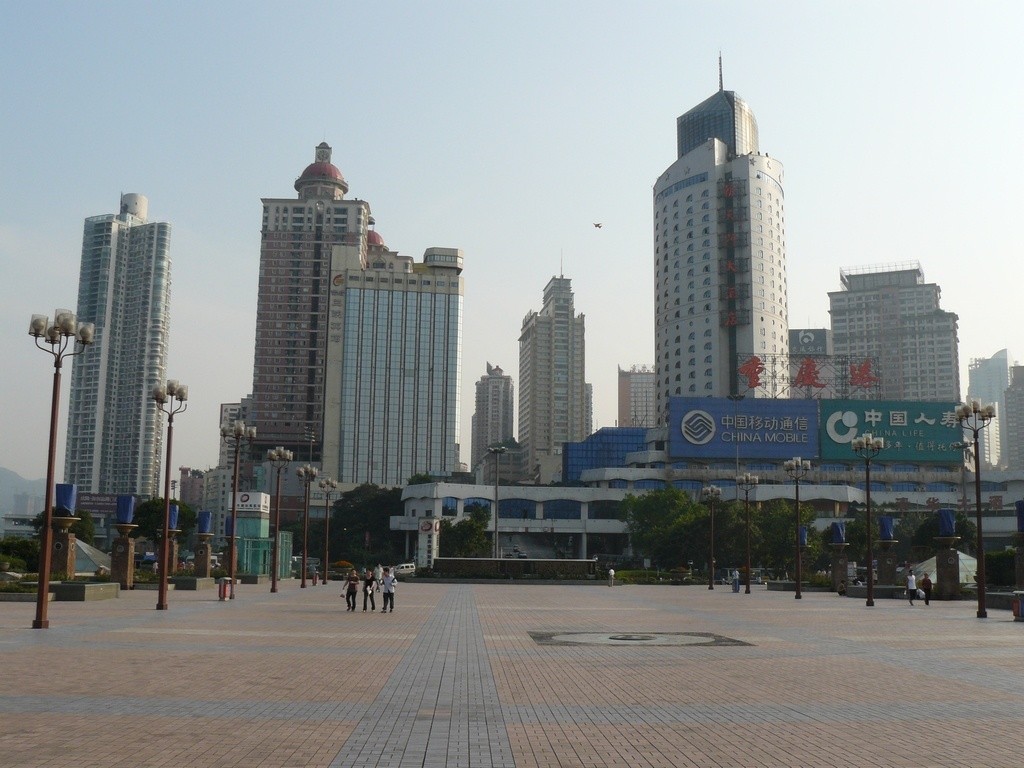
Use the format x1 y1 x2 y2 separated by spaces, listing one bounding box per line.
1012 592 1024 621
312 572 319 586
218 577 231 601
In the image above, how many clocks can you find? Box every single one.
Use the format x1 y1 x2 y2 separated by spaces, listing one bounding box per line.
318 150 329 160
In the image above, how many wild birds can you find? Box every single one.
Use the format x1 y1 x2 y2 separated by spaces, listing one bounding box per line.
593 222 602 228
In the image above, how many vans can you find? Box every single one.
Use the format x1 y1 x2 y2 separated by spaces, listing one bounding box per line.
392 562 416 578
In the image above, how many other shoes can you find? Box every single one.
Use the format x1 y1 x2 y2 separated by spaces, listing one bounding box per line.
909 601 913 605
363 609 366 612
390 608 392 612
381 609 387 613
372 609 375 612
351 608 355 611
347 606 351 611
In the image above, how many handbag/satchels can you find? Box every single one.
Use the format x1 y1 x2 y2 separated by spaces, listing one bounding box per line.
903 590 907 595
339 590 346 599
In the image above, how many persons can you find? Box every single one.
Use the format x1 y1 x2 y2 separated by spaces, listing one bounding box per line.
923 573 931 605
732 568 739 582
379 568 397 613
609 567 614 587
153 558 219 573
906 570 917 606
343 569 360 611
873 570 878 583
373 563 396 593
837 580 845 595
853 576 865 585
362 569 376 612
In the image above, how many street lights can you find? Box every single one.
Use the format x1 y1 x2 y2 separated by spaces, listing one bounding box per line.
266 445 294 594
26 307 95 629
851 433 884 607
150 378 190 611
735 472 759 595
218 419 257 602
487 446 508 558
954 398 997 619
701 484 722 591
295 462 319 589
782 456 811 600
318 476 339 586
